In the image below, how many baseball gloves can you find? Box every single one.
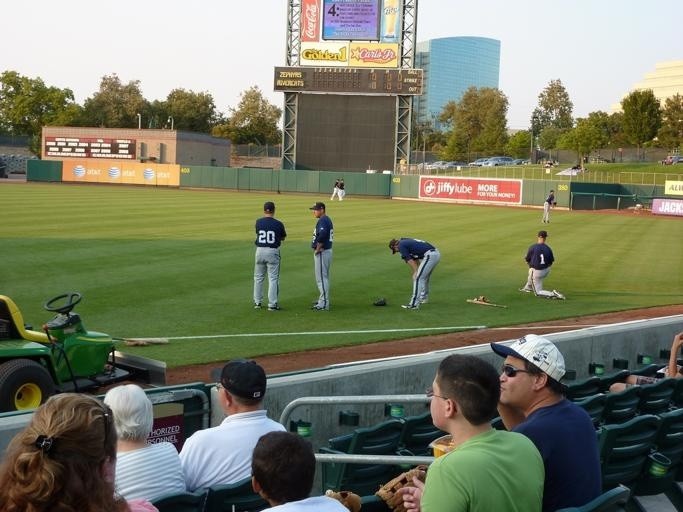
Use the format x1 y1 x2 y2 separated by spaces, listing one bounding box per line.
325 490 363 512
479 296 490 303
376 464 428 512
553 201 557 205
374 298 385 305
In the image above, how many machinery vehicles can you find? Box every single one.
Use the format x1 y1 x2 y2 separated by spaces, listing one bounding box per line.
0 290 170 414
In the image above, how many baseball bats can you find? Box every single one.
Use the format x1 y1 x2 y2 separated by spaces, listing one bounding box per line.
466 299 508 308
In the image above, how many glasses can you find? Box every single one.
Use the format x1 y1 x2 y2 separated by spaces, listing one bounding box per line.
426 388 457 413
502 364 536 377
81 394 109 450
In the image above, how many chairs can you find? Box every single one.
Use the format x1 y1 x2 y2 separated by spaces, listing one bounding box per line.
0 295 54 344
149 358 683 512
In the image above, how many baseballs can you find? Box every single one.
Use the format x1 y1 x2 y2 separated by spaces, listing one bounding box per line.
143 169 154 180
108 167 119 179
75 166 85 177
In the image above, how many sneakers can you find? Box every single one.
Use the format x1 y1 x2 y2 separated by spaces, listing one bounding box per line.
312 302 329 311
553 290 564 300
518 288 530 293
254 302 261 308
401 298 427 310
268 306 279 311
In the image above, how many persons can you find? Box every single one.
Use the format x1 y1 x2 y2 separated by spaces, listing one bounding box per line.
308 201 334 311
632 198 644 216
253 201 287 311
519 230 565 300
544 159 581 173
252 431 350 512
491 333 602 512
608 332 683 393
397 354 545 512
104 384 186 500
552 194 558 209
541 188 555 224
0 392 159 512
389 237 441 310
339 178 345 198
672 156 678 166
179 359 287 491
331 178 344 201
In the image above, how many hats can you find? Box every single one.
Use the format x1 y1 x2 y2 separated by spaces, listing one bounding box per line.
264 202 275 212
491 334 566 381
220 361 266 398
309 203 324 210
389 239 398 254
538 231 547 238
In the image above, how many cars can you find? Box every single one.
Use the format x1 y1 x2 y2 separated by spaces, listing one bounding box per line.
661 155 683 165
416 156 531 169
